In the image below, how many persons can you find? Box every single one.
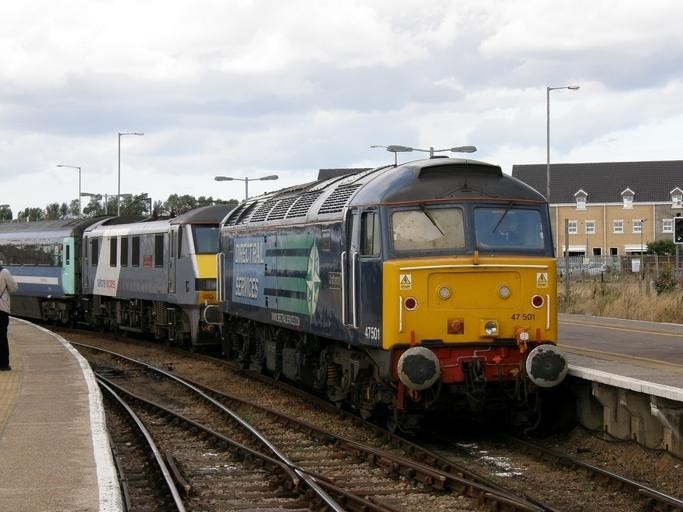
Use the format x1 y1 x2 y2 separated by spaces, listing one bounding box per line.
486 213 522 245
0 258 18 371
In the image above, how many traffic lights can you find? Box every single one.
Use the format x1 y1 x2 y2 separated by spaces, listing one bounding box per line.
673 217 683 245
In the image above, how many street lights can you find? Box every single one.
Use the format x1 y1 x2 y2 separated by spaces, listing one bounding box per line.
81 194 132 215
546 86 580 203
215 175 278 201
118 132 144 216
58 164 81 219
371 145 477 166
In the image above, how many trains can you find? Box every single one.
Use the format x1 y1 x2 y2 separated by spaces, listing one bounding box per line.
0 155 569 436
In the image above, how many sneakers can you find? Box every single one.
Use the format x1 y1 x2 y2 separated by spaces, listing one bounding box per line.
0 365 12 371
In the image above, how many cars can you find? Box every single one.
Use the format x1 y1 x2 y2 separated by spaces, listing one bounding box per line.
558 261 609 282
